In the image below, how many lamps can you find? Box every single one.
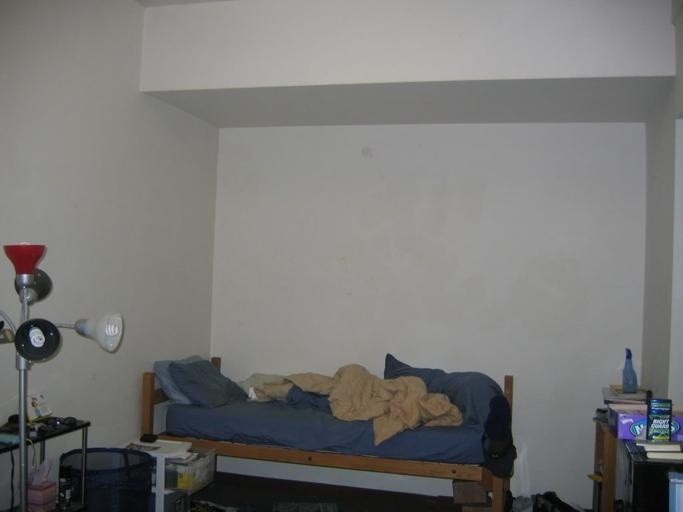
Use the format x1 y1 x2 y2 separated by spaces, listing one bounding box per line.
0 245 123 512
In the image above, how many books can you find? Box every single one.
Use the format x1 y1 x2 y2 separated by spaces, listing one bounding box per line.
636 439 683 460
593 384 647 423
26 392 53 423
124 439 198 464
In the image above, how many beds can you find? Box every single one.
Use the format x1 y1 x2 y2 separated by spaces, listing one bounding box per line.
140 356 514 512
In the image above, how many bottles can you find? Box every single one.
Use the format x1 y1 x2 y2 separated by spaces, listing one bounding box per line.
622 344 637 393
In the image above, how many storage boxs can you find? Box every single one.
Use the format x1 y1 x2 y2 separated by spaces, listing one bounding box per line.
150 491 190 512
152 447 216 496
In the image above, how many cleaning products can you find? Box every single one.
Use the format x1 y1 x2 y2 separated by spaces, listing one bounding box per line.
623 348 638 392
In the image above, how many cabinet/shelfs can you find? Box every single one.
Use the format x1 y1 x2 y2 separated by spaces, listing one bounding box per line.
591 411 683 512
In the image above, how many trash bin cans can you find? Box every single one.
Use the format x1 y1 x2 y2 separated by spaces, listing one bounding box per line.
59 447 152 512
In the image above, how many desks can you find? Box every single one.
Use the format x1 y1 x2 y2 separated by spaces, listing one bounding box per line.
0 417 91 512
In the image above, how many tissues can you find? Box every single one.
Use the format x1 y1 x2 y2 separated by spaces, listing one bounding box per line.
28 455 59 511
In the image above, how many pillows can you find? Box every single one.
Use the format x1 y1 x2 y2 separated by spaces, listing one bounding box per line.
169 359 248 408
153 355 202 404
